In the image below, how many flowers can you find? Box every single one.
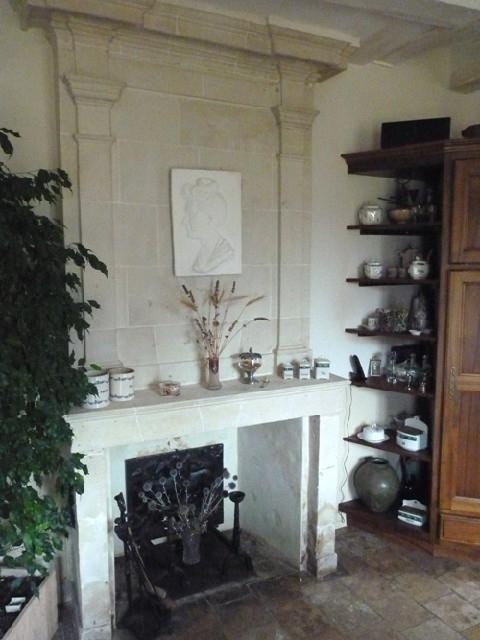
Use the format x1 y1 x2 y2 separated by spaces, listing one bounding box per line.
137 461 239 537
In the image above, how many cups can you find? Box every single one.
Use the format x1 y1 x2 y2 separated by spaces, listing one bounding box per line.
80 366 135 409
363 426 384 441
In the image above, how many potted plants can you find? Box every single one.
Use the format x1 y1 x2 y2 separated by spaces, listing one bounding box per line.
178 277 272 391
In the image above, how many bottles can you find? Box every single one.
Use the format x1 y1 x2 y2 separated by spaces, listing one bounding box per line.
408 256 429 280
360 206 384 226
364 263 384 278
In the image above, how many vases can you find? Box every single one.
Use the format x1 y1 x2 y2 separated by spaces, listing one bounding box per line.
180 528 203 566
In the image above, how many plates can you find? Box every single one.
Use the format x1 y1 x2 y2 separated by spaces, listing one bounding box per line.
357 432 390 443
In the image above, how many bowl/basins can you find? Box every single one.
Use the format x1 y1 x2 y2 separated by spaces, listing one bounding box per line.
390 209 411 221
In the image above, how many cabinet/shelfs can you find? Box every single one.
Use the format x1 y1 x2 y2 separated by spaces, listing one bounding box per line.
439 157 480 549
340 144 442 549
